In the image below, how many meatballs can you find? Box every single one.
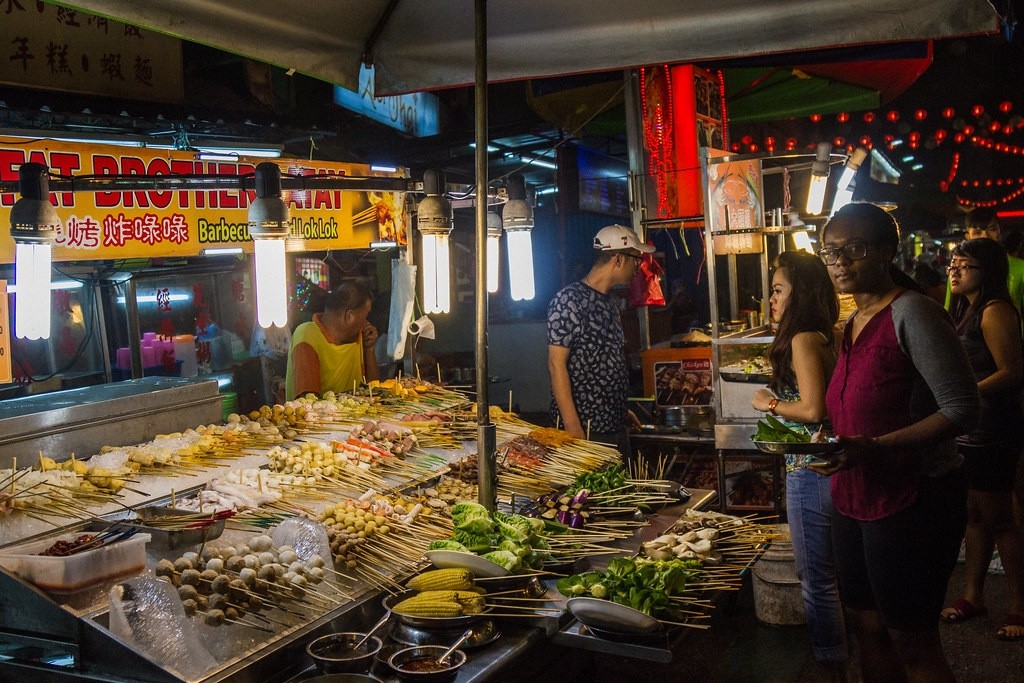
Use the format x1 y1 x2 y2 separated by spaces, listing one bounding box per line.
14 391 432 628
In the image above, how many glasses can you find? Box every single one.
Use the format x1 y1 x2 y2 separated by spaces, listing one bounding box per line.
817 242 884 266
621 252 643 265
945 265 980 274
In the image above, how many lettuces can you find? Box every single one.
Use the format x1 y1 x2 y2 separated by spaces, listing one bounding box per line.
427 502 551 573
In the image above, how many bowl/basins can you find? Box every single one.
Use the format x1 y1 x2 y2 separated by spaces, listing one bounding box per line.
222 392 239 422
388 645 466 682
307 632 382 674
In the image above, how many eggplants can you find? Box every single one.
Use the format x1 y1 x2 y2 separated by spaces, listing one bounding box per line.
519 489 590 530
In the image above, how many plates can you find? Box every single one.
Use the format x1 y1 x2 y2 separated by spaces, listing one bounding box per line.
426 550 512 582
385 587 496 622
565 597 661 635
750 439 843 455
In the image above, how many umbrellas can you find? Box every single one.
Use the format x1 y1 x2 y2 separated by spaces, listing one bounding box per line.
43 0 1007 512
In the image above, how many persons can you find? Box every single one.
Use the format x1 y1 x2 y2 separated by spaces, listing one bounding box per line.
804 201 987 683
749 249 872 682
939 236 1023 645
285 279 380 403
546 223 657 476
942 207 1023 343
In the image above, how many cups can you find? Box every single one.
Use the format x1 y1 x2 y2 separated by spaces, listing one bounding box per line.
746 311 759 329
115 332 172 368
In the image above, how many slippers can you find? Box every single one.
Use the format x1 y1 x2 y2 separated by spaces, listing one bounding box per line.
997 614 1024 641
941 598 987 623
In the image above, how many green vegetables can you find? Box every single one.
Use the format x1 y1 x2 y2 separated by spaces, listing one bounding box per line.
555 557 687 622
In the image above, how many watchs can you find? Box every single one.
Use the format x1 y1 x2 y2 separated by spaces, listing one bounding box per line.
767 398 781 416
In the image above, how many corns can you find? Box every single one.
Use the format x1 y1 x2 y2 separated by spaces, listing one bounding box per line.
391 567 488 618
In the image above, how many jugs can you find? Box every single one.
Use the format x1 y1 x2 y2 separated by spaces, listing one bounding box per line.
173 334 199 378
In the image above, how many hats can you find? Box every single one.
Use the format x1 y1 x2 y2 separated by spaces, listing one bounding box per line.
593 224 656 254
